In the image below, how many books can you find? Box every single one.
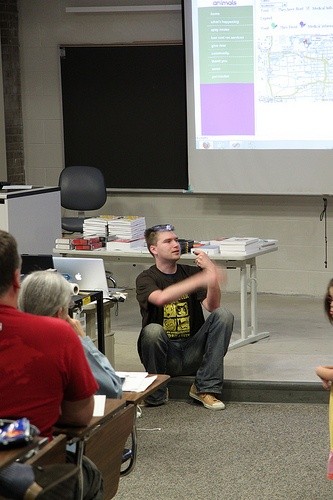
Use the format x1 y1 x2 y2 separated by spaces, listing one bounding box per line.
191 237 279 256
54 215 146 249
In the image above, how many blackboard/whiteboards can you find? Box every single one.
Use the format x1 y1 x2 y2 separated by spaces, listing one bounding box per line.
57 40 189 194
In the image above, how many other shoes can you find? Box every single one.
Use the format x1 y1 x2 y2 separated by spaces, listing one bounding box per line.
139 399 148 407
189 382 225 410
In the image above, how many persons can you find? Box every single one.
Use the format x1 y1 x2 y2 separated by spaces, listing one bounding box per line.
0 463 79 500
315 276 333 480
0 229 105 500
17 270 126 400
133 223 236 412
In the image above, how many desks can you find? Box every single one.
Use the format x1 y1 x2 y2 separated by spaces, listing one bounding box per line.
0 373 171 500
52 247 278 350
0 185 62 254
70 290 105 355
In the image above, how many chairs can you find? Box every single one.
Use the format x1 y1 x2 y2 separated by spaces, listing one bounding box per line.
59 166 118 316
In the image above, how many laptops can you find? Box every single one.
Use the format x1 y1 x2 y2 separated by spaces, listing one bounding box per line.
52 256 124 299
